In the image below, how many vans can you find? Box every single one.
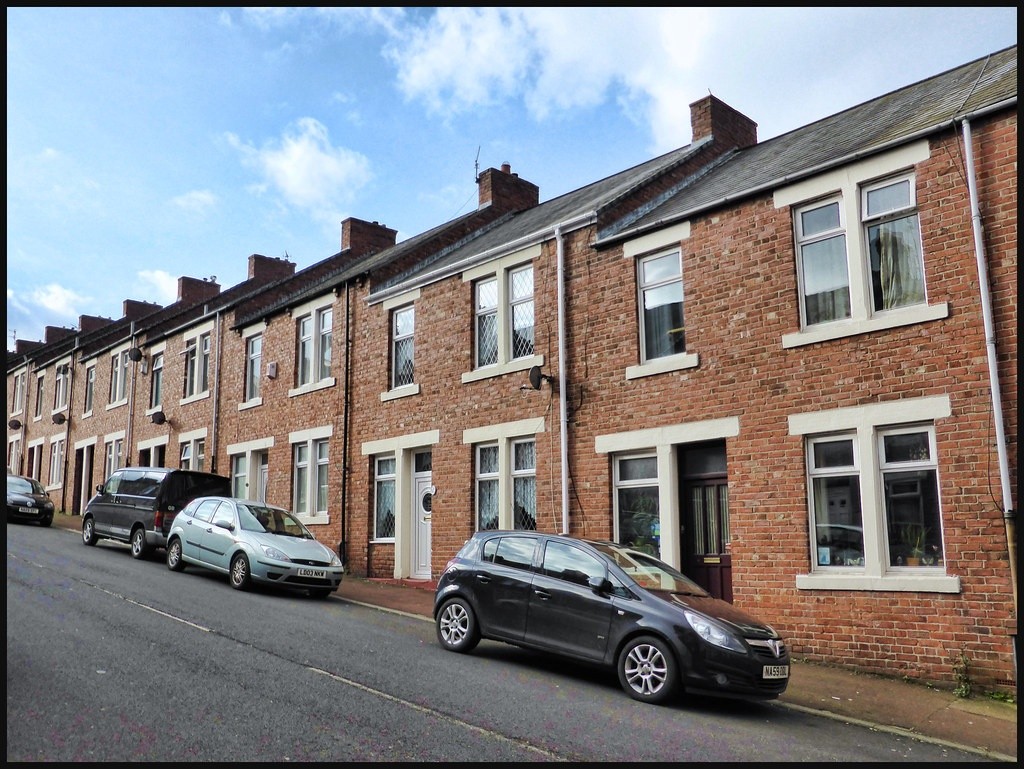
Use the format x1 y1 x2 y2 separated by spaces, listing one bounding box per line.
82 467 232 560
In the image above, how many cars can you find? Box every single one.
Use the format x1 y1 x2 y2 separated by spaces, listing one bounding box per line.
167 496 345 601
433 530 792 704
7 474 55 526
